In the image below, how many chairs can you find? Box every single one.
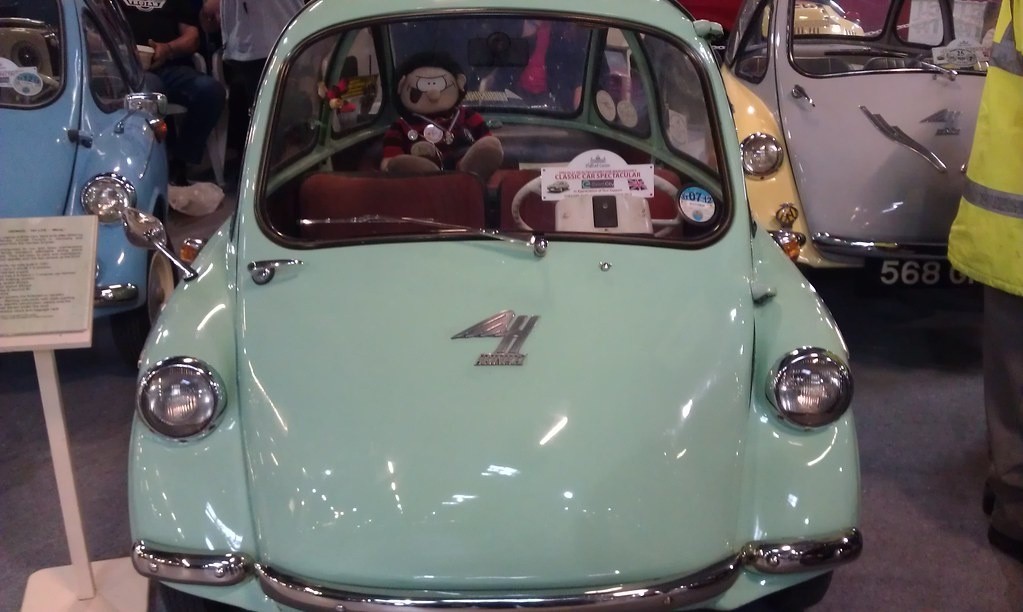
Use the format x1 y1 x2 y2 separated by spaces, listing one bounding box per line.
303 172 486 240
863 57 921 70
498 170 683 235
796 59 855 75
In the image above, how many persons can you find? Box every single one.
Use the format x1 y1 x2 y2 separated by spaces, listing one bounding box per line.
946 0 1023 562
111 0 225 187
201 0 591 191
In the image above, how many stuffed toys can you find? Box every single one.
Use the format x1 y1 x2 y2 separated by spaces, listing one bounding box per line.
377 50 505 182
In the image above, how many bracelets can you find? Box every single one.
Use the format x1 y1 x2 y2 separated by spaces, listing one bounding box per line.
167 43 176 56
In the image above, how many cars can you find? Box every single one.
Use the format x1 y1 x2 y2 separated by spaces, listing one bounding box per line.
1 0 179 372
122 1 866 612
332 2 821 278
725 0 1002 291
